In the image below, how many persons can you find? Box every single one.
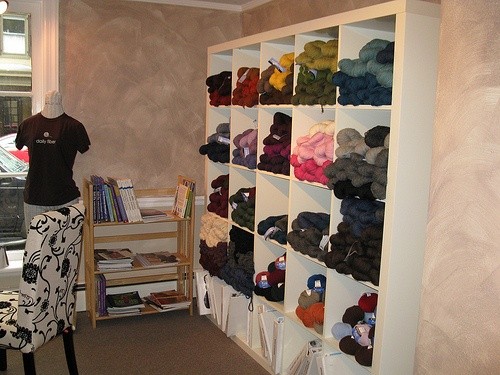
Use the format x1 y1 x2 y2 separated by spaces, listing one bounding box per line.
15 89 91 233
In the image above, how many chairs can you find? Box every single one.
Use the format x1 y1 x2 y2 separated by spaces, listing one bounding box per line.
0 205 85 375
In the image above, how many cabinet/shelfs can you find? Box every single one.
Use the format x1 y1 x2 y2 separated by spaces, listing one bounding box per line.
201 0 441 375
77 176 197 328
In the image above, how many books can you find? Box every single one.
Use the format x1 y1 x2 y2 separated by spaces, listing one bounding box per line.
106 291 145 316
95 274 107 316
95 248 134 271
142 289 191 311
140 179 195 222
89 174 142 223
136 252 180 267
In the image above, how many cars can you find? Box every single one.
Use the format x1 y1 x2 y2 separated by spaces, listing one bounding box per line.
0 133 29 236
